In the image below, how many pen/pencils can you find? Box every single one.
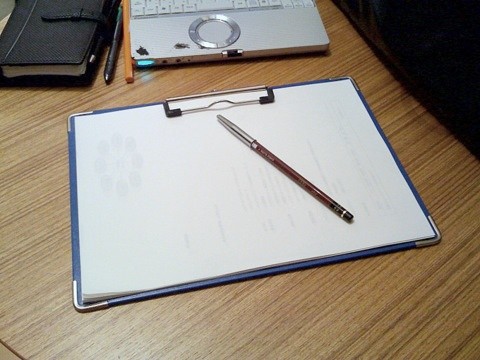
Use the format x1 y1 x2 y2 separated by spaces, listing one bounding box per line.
103 4 124 83
215 113 355 224
121 0 134 83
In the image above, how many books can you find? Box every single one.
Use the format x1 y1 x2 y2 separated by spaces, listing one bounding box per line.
0 0 122 87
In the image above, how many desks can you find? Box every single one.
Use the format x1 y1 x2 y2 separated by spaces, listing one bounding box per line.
0 1 480 360
